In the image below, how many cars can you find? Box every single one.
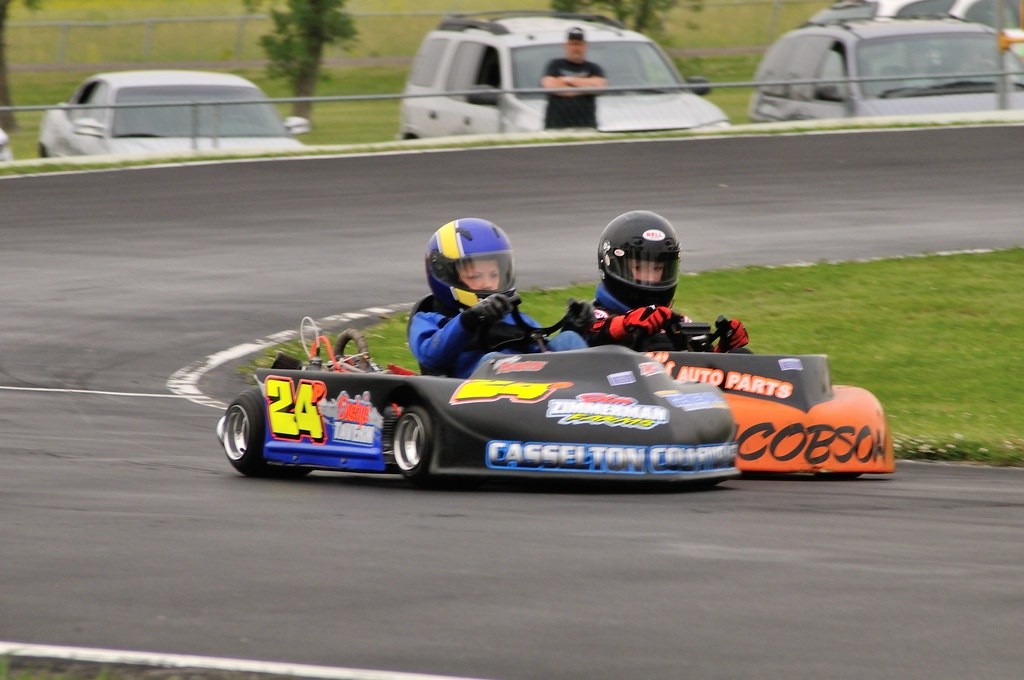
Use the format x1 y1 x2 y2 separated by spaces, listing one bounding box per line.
36 66 312 159
879 0 1024 58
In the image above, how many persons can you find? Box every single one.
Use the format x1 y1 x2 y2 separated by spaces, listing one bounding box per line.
540 27 608 130
405 218 595 381
560 208 754 355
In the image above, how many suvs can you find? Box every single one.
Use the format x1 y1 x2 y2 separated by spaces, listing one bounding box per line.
746 11 1024 126
392 9 736 143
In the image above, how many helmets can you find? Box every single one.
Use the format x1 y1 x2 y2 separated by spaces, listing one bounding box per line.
425 217 516 310
597 210 681 308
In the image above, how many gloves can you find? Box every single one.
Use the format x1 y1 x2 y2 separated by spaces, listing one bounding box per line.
714 314 749 354
460 293 512 329
609 305 671 340
561 302 594 340
